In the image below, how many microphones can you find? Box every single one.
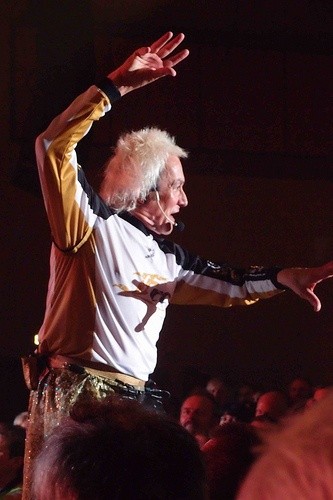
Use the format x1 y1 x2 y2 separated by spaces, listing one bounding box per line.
154 187 178 227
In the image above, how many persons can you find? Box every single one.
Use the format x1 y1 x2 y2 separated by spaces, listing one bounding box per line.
181 374 333 500
32 398 205 500
241 398 333 500
0 424 27 500
22 31 333 500
15 411 27 430
